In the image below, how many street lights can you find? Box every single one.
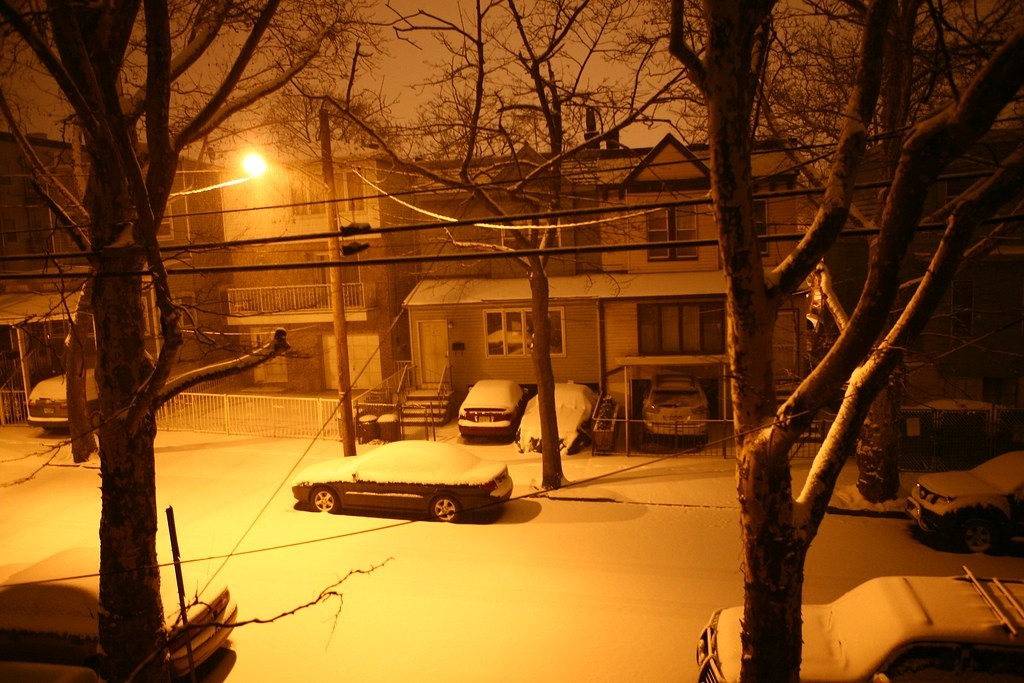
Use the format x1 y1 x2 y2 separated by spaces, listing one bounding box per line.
243 149 357 459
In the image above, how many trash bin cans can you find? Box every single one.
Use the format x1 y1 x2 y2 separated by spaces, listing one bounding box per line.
379 413 401 442
359 414 378 444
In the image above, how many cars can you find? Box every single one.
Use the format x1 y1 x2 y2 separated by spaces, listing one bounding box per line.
640 374 710 448
289 438 513 524
903 450 1024 556
26 364 101 434
0 545 238 683
457 378 530 439
515 383 598 455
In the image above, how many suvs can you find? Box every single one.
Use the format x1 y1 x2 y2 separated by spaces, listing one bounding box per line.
695 564 1024 683
